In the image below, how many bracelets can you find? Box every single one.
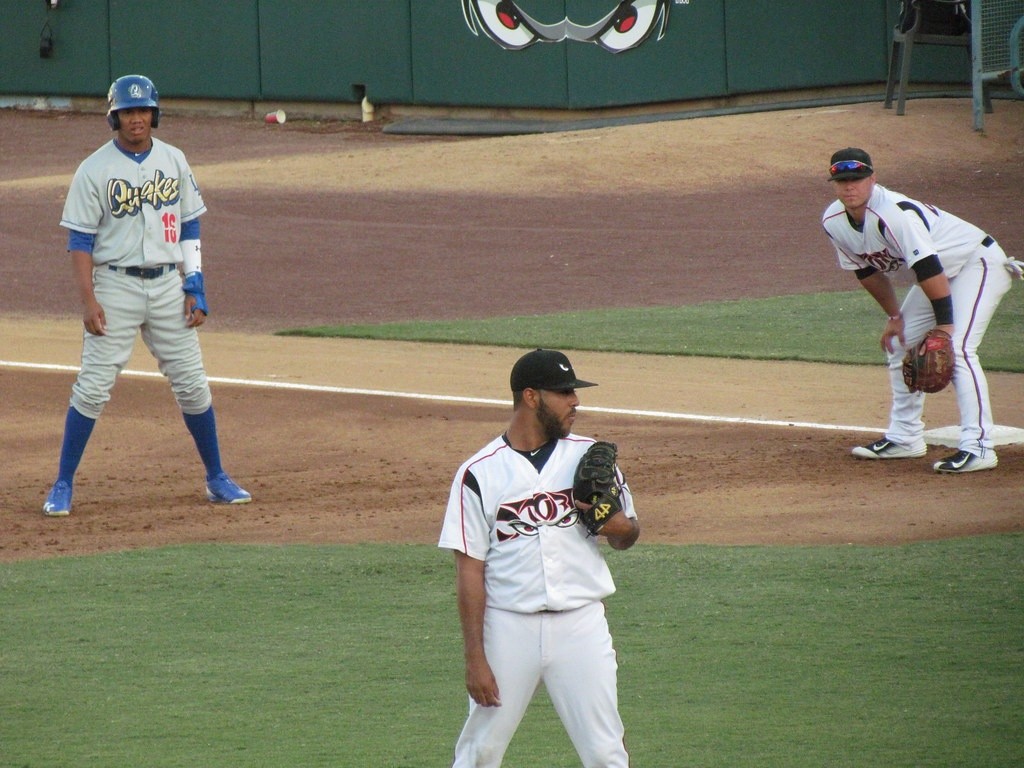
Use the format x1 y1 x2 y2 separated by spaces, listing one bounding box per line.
887 314 902 321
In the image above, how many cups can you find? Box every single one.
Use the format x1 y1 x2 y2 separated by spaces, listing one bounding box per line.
265 109 286 124
361 98 375 122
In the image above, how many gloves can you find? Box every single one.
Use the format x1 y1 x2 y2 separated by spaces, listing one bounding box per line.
1003 256 1024 281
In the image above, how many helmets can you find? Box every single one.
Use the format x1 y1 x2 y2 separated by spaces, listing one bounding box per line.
106 74 159 130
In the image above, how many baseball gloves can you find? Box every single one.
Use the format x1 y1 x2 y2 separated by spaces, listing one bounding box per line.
572 441 624 537
901 328 956 398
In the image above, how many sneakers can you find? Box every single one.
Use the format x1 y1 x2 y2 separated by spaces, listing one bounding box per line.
205 473 252 504
851 433 928 460
933 450 999 474
43 479 73 517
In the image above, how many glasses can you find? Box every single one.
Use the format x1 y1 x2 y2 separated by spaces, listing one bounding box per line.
831 160 863 173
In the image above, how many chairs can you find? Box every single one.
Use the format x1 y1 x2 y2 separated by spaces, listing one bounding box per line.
883 0 994 116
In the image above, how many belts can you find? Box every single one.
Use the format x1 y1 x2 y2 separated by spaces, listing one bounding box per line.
981 236 994 248
109 264 175 279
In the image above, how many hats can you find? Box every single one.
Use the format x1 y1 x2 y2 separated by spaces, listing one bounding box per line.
826 146 874 181
510 348 598 390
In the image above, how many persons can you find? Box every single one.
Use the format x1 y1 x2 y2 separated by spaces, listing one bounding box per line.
40 73 254 518
436 347 640 768
820 145 1024 476
